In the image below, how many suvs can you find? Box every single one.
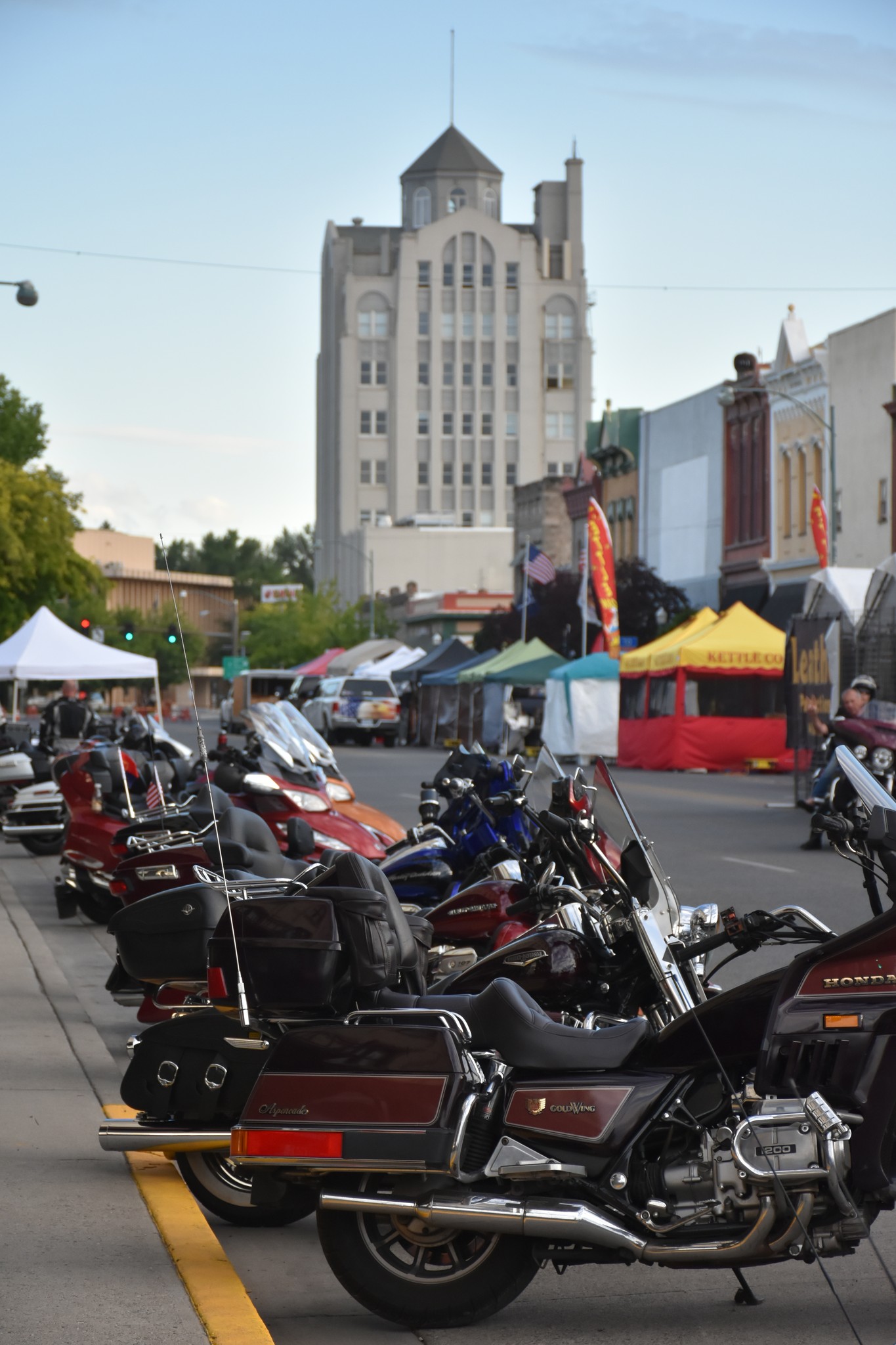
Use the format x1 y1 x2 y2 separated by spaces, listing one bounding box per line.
298 674 403 747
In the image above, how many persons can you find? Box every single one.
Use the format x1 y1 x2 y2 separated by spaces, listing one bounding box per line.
38 681 97 748
798 689 864 813
800 674 877 851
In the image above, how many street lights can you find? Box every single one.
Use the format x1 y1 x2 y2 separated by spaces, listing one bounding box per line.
716 385 837 568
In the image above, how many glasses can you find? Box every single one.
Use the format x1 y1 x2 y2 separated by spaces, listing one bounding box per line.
858 687 871 695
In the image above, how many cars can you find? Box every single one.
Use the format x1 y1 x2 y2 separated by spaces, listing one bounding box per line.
285 673 327 711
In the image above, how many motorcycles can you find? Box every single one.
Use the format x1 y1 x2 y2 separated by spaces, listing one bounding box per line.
0 671 834 1230
219 743 895 1329
797 717 896 854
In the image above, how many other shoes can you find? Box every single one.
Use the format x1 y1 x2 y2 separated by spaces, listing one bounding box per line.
800 838 822 850
799 799 816 813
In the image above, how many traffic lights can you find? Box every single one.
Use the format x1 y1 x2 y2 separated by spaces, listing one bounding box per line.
81 619 90 635
125 622 134 641
168 625 177 644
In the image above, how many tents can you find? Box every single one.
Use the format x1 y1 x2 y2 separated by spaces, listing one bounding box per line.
617 601 815 774
0 605 163 730
542 652 621 768
295 635 565 759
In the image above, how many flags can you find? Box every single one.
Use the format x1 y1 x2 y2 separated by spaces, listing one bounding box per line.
810 488 832 569
524 544 557 586
584 500 624 659
118 751 139 779
145 773 163 809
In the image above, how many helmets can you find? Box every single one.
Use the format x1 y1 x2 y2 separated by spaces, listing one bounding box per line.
851 673 877 698
